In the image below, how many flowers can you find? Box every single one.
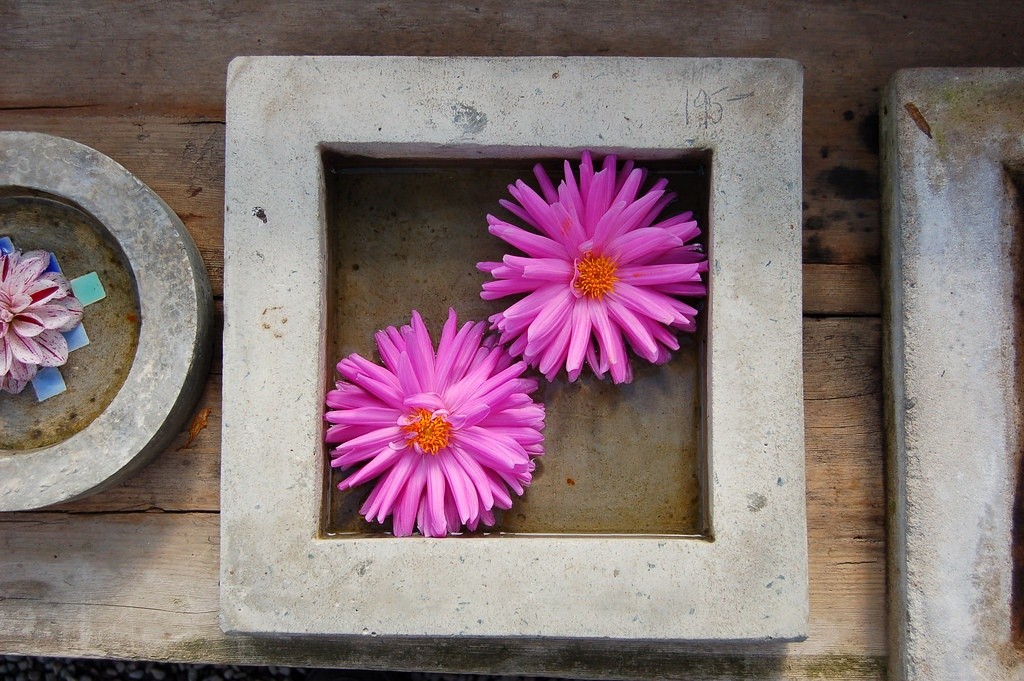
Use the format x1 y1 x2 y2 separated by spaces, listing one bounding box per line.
0 250 83 396
475 149 708 385
323 308 546 538
